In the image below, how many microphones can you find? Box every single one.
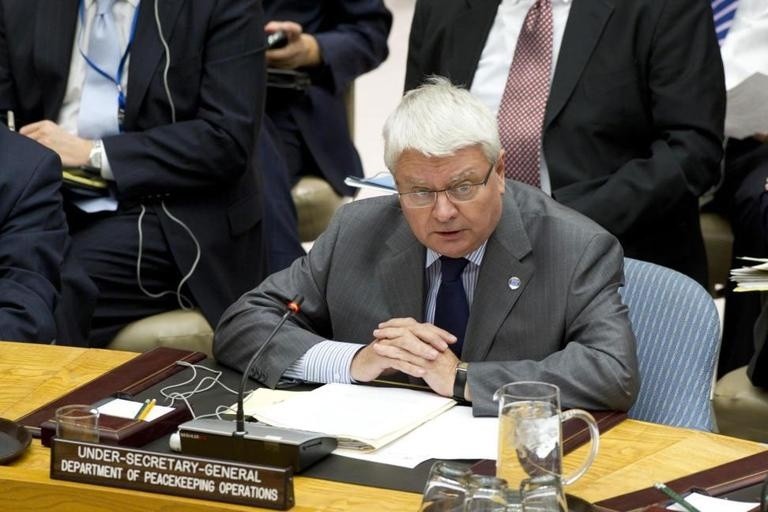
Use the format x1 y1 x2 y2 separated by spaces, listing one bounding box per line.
178 294 338 476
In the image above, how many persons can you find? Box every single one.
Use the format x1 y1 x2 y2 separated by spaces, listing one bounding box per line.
1 0 768 410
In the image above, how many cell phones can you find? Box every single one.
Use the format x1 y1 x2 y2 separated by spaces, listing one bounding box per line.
268 29 288 49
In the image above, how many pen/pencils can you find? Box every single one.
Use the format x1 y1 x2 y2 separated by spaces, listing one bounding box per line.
656 482 701 512
134 399 157 423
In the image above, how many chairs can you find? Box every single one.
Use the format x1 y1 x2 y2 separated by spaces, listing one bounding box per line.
289 77 354 241
121 306 218 361
697 209 736 295
618 257 719 435
715 360 768 441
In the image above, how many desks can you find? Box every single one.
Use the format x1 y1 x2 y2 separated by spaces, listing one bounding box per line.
0 338 767 512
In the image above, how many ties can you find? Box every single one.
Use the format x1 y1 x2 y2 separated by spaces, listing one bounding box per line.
71 0 124 216
490 1 558 191
431 254 472 364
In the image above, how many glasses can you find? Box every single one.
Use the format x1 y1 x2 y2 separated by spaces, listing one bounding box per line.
389 172 492 210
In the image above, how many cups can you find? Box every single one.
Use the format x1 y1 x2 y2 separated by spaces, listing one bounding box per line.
55 402 100 444
490 375 602 498
416 459 571 512
